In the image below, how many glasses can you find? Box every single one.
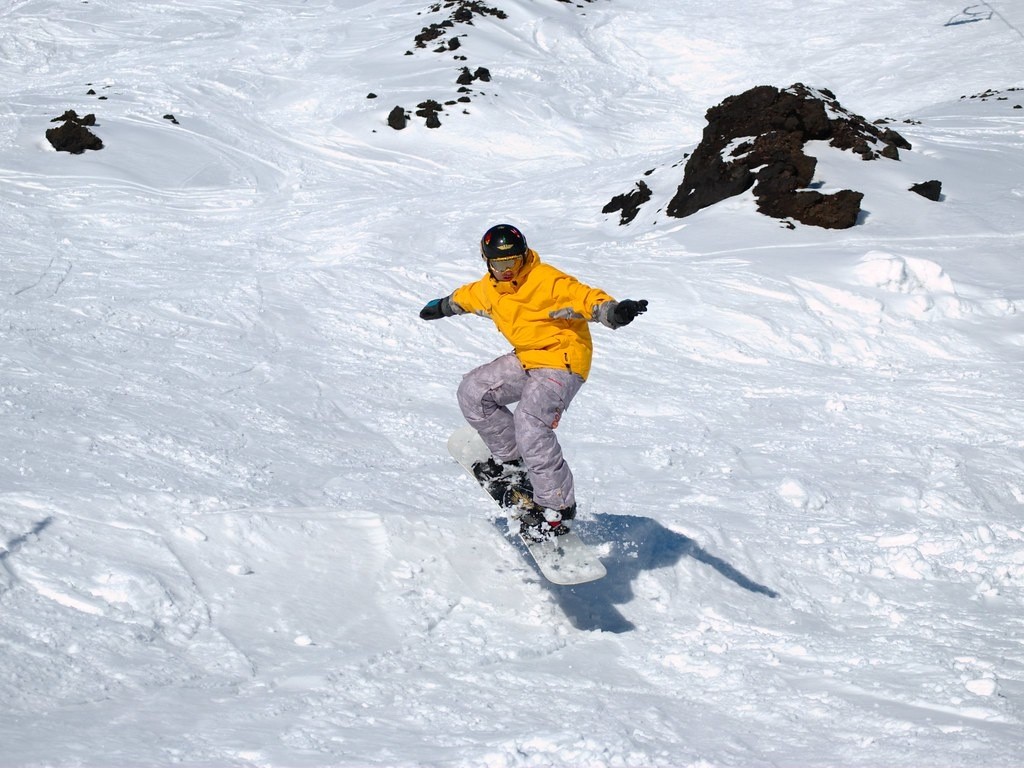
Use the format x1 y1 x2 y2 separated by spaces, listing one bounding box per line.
488 257 522 271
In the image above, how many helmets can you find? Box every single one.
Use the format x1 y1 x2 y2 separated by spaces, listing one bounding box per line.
481 225 528 261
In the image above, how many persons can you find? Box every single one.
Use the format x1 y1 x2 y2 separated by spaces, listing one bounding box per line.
419 224 648 542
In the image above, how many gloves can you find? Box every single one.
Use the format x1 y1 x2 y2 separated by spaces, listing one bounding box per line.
608 299 650 328
419 296 451 321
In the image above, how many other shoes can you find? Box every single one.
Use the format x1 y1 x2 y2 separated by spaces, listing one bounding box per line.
473 457 523 483
519 502 577 527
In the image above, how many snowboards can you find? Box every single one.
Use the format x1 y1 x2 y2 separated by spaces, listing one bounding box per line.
445 422 612 590
